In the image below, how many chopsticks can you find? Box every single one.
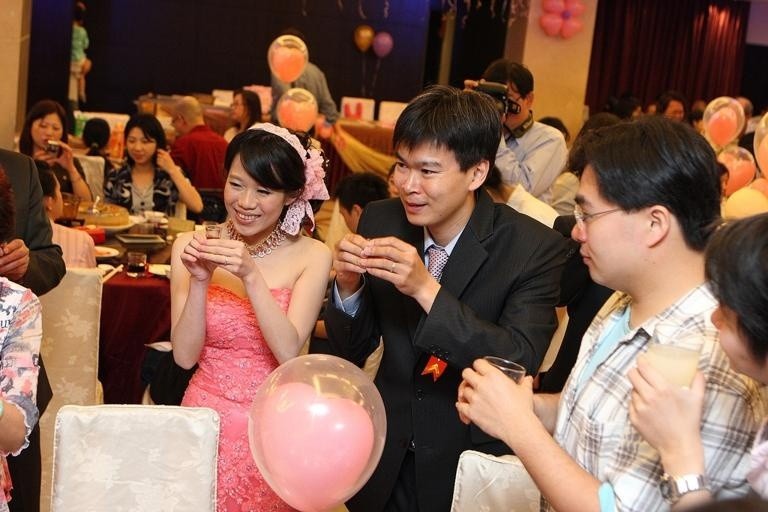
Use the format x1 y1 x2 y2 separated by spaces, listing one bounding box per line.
101 264 124 283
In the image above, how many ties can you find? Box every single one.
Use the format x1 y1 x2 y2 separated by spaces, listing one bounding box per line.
427 247 449 283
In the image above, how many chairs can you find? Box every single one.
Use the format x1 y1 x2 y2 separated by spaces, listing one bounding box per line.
50 403 219 510
39 269 102 511
339 97 374 121
77 110 129 131
325 199 349 254
377 101 408 125
73 154 105 203
450 450 541 510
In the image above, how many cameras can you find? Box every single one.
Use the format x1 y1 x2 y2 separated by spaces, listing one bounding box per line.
470 80 524 118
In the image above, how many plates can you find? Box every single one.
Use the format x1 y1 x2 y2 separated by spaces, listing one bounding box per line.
149 264 171 276
95 246 120 259
97 216 139 231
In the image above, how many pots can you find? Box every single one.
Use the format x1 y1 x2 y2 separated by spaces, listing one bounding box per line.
61 192 84 219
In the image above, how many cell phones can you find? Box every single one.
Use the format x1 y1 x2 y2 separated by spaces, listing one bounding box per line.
41 142 64 160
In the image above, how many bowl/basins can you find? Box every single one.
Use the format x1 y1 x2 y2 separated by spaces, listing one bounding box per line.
144 211 165 222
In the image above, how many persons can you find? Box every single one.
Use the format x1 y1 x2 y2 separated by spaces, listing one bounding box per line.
69 2 92 110
1 149 67 512
271 31 338 132
19 59 768 512
0 165 44 512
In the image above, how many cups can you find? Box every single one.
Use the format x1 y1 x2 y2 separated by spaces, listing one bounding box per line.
127 252 148 278
205 226 223 239
485 356 526 386
45 140 58 153
650 324 705 391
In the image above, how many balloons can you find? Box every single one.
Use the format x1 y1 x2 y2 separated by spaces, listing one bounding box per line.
374 31 392 58
355 25 374 51
267 34 309 82
540 0 587 37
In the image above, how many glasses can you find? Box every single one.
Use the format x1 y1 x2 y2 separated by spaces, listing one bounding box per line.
170 116 178 128
572 204 635 232
231 103 244 109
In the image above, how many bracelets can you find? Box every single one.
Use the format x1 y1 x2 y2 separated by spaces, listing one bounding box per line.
0 400 4 417
